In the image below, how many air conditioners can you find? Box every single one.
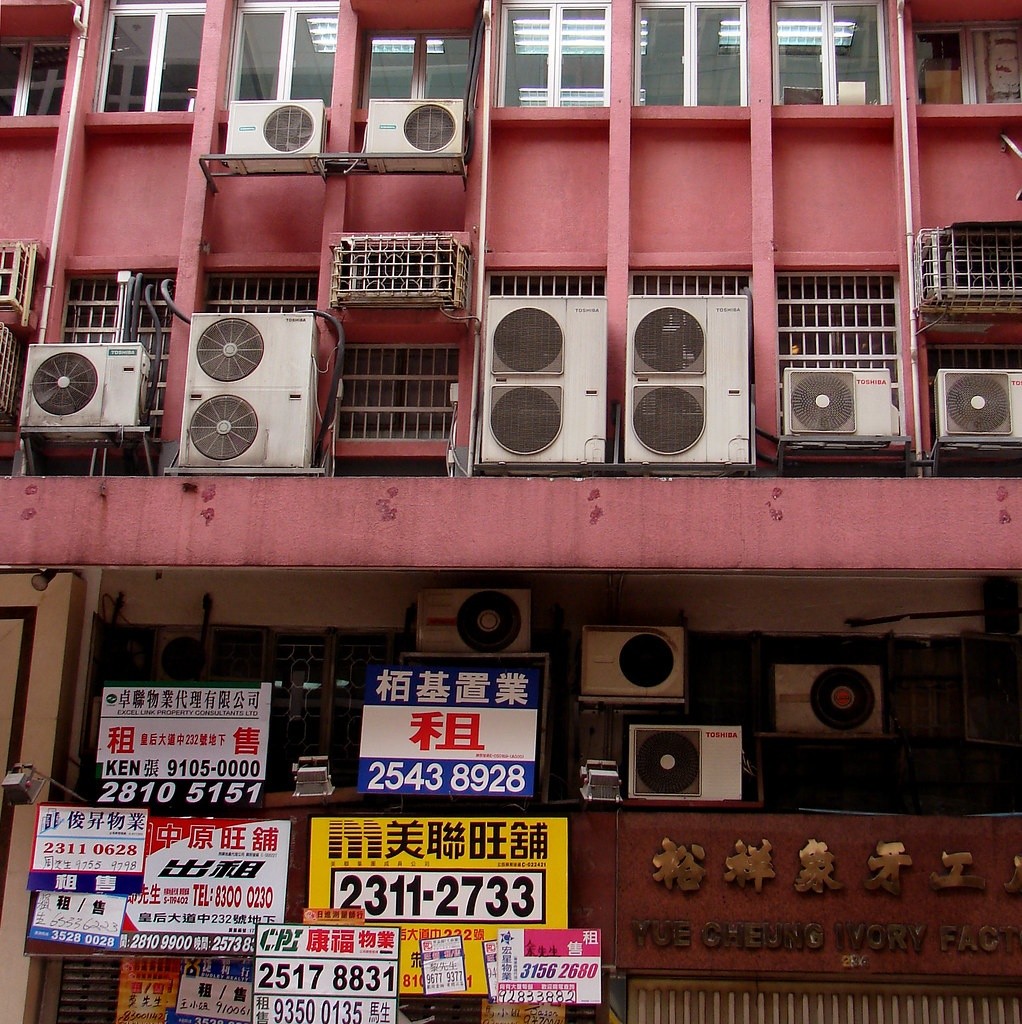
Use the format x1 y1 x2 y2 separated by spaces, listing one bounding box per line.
414 586 888 802
0 98 1022 482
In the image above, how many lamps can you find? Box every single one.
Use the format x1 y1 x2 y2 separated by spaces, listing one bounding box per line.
1 763 46 804
